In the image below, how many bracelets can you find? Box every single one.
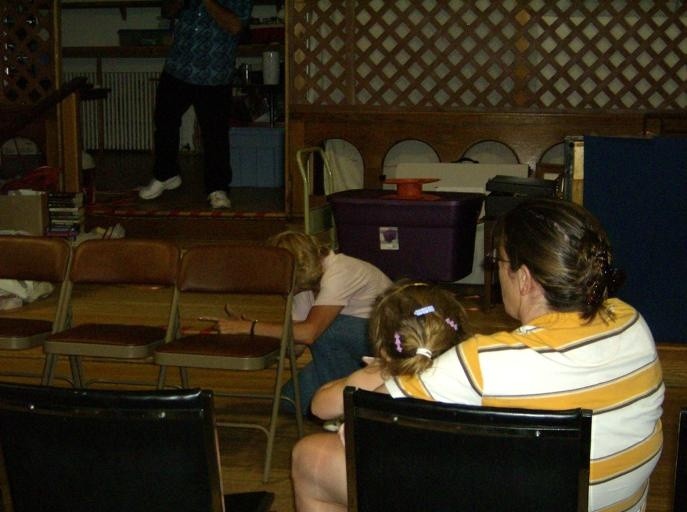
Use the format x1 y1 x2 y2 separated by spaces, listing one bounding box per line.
251 319 258 335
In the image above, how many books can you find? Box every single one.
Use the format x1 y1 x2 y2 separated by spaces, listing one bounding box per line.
43 190 86 240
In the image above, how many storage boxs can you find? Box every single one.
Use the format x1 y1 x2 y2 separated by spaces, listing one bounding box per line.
326 178 486 282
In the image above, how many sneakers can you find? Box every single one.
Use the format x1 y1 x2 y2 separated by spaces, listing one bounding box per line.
139 173 183 200
208 190 231 210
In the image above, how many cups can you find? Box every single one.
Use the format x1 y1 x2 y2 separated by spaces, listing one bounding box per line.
262 51 279 84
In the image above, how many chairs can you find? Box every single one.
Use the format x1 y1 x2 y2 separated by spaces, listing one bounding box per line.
46 235 187 389
149 242 303 485
343 385 593 511
2 233 80 390
1 377 282 510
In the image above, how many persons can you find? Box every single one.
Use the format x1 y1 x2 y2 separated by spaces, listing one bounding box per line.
308 282 472 423
290 192 667 512
137 0 249 209
197 232 393 433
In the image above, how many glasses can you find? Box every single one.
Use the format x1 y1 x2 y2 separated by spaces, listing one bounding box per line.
482 248 507 271
